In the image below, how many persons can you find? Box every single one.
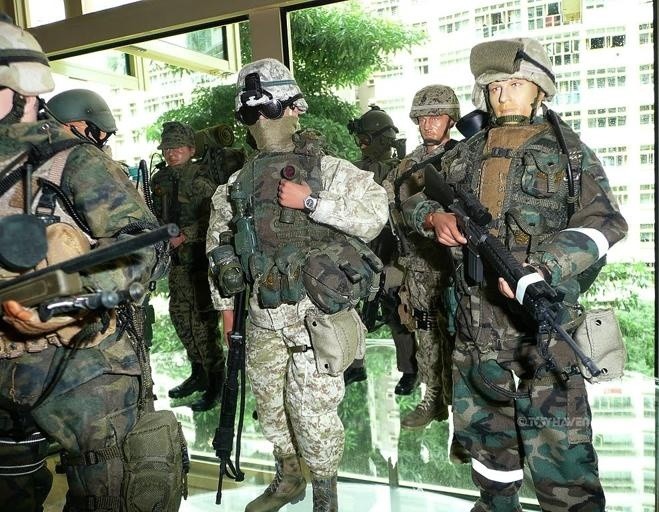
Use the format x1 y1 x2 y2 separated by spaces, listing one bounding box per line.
343 103 420 396
1 20 161 511
148 121 226 412
204 57 390 512
381 85 463 430
399 37 628 512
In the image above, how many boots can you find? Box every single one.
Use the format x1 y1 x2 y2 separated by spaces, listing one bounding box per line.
401 385 447 432
169 360 225 411
309 469 337 512
244 452 305 512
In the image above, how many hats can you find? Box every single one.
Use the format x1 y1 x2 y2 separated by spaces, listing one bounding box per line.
156 121 197 151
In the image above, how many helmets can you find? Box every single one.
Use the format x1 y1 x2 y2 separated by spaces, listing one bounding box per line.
1 23 54 95
44 89 117 136
470 37 553 109
346 107 396 138
409 85 461 125
234 59 307 111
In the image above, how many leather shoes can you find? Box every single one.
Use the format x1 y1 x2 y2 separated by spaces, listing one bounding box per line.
344 366 366 385
394 372 416 394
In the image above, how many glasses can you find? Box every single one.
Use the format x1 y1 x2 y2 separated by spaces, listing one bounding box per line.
233 95 302 125
353 125 391 147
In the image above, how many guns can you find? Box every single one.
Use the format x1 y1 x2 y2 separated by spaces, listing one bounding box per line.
360 223 397 334
210 183 262 504
423 163 601 377
0 214 179 322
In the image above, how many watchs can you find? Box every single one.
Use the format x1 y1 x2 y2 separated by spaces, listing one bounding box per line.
303 192 318 212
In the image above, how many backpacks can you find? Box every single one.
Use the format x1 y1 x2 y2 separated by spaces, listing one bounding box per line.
187 148 242 201
